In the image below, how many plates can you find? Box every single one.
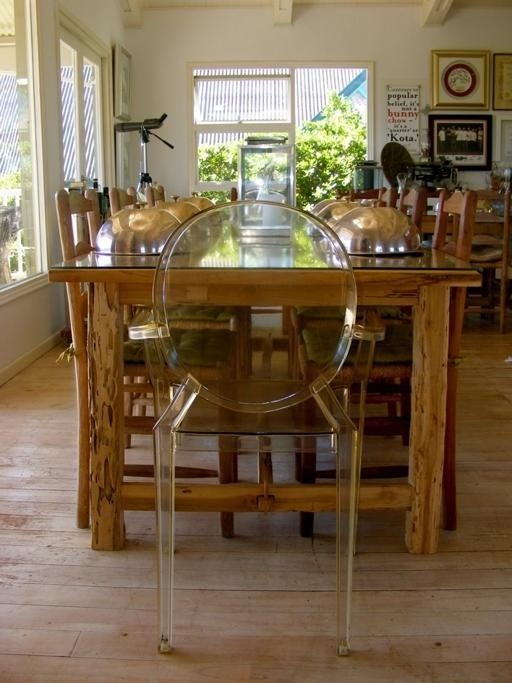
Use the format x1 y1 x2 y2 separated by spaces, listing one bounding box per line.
347 248 425 257
92 250 187 255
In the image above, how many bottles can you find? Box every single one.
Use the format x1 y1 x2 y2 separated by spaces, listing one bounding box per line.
104 186 111 216
93 178 104 215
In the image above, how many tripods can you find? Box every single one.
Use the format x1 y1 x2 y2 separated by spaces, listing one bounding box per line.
136 128 174 193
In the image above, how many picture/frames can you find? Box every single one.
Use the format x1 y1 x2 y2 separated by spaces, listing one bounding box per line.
112 44 132 122
428 47 509 171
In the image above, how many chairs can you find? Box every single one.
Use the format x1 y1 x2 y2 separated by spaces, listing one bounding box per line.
129 200 385 655
54 183 254 539
454 188 511 334
282 179 477 537
